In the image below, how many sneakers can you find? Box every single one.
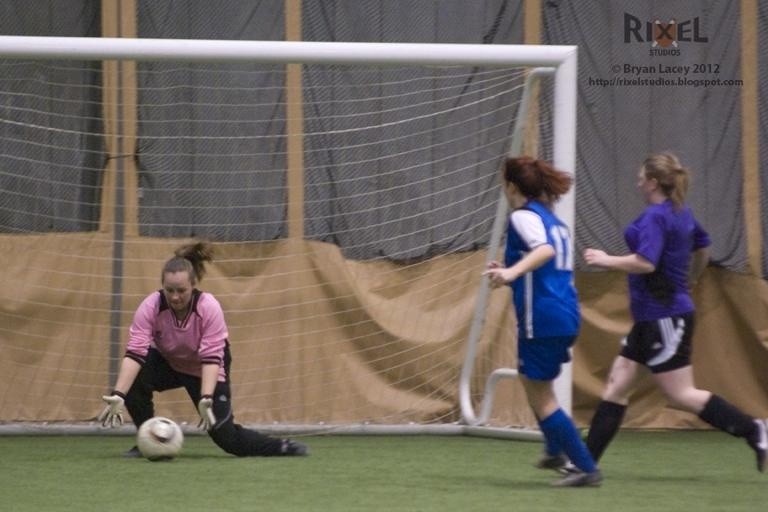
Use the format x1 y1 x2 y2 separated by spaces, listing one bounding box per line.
753 417 767 469
280 438 309 456
124 446 141 458
537 452 604 487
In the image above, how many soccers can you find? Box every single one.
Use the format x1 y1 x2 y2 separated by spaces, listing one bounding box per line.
135 416 183 463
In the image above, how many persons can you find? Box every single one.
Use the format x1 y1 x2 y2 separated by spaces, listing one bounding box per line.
558 151 765 480
479 157 605 488
99 241 309 456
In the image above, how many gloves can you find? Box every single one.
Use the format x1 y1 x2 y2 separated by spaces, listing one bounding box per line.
196 395 216 430
98 391 127 429
